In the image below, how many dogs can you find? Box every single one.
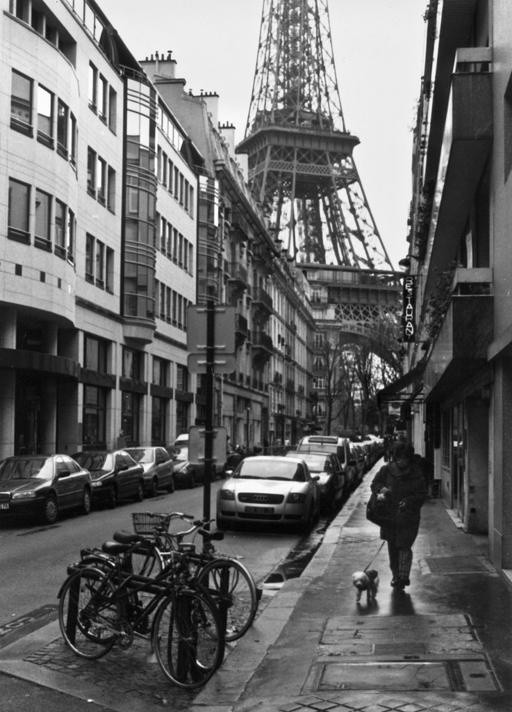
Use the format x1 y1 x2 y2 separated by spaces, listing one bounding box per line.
350 567 379 603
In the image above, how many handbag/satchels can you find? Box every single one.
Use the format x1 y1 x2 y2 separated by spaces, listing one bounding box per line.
366 490 407 530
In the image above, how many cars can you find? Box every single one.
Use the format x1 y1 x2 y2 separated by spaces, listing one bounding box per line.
285 433 384 505
122 446 176 497
166 445 215 490
71 449 145 510
0 453 93 523
216 455 321 531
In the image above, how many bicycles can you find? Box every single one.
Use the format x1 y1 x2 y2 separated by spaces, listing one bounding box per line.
57 511 258 688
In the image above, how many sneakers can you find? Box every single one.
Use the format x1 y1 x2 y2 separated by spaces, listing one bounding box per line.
391 575 410 588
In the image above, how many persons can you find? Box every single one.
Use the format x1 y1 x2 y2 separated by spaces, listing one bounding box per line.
369 440 428 589
